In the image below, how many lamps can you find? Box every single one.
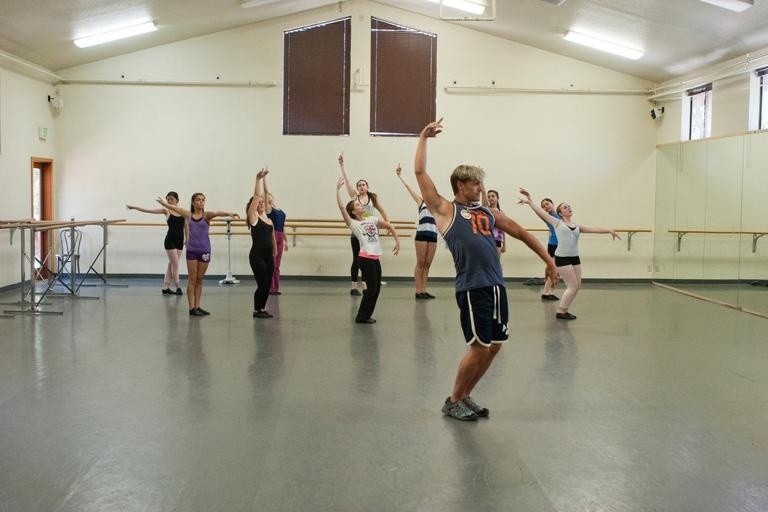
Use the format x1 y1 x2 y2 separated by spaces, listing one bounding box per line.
650 105 665 120
69 6 160 52
437 0 487 19
563 18 643 64
704 0 754 15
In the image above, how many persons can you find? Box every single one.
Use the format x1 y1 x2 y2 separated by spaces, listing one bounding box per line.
396 162 439 299
261 165 289 296
337 151 394 296
518 185 622 320
414 116 561 421
125 191 190 295
154 192 240 315
335 176 401 323
481 185 507 258
516 196 562 301
245 167 278 319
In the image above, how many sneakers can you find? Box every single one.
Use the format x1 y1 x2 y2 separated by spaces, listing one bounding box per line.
189 307 204 316
424 292 436 299
196 307 210 316
541 294 560 301
460 396 490 418
261 310 273 318
175 287 183 295
350 289 362 296
161 287 177 295
556 311 576 320
415 292 429 300
355 318 377 324
252 311 269 319
441 395 479 422
268 291 282 295
363 289 367 295
